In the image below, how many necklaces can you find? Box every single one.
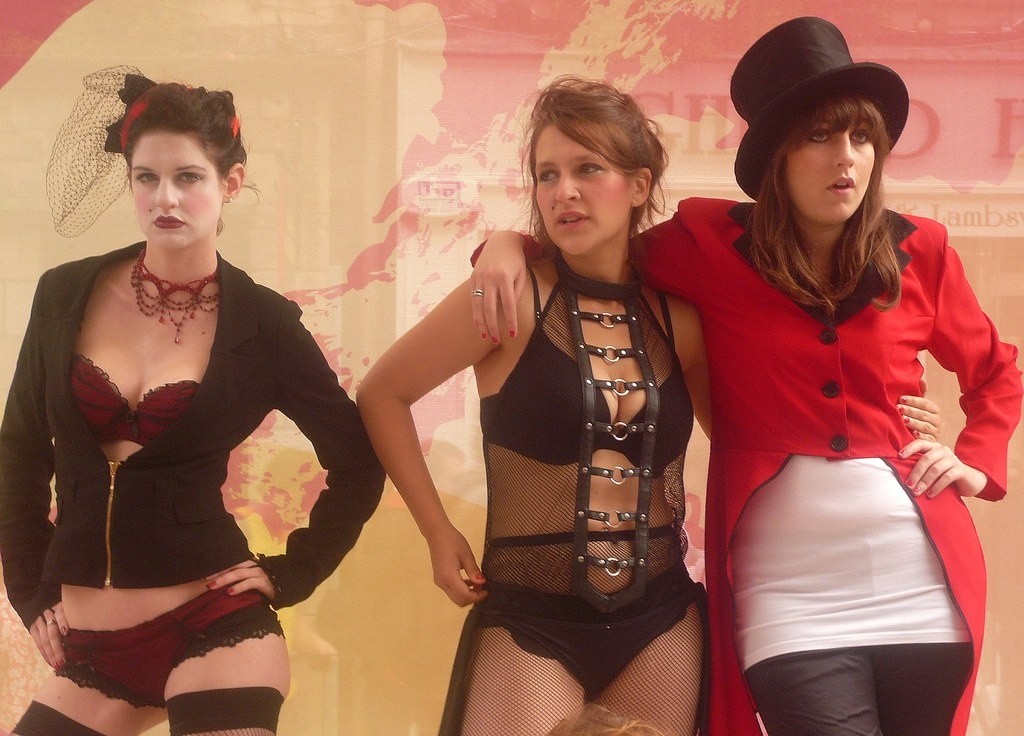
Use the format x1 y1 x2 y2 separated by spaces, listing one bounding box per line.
131 249 221 343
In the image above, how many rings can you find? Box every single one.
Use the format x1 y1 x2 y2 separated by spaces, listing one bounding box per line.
46 618 57 625
472 290 484 296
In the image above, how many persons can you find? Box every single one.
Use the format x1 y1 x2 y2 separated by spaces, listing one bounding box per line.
471 15 1024 736
356 73 942 736
0 64 385 736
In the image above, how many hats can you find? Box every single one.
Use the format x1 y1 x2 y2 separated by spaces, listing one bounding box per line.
730 17 909 202
